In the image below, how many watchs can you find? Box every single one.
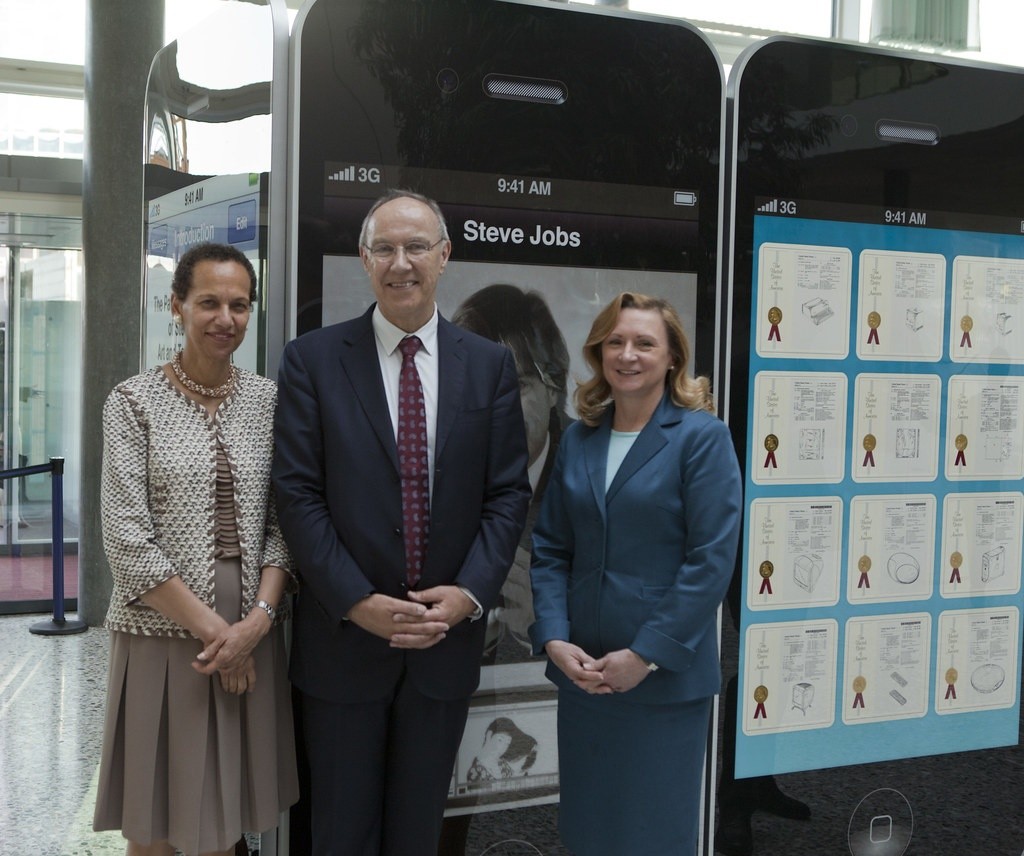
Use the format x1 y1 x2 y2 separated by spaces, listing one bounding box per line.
253 599 279 624
638 655 659 672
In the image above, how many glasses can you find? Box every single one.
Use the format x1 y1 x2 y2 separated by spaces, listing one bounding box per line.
359 237 448 260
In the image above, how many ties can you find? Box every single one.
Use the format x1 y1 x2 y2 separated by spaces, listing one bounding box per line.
396 335 431 587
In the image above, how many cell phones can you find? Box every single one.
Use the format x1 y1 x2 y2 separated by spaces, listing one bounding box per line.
133 0 1024 856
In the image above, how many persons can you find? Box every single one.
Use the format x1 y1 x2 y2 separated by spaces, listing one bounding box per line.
451 284 579 664
528 292 743 856
93 243 299 856
270 188 533 856
468 717 537 783
